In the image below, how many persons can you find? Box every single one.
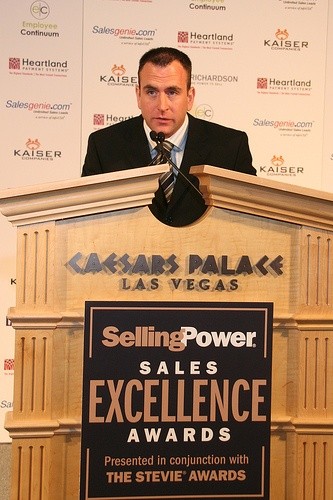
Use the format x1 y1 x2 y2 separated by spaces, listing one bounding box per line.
80 46 259 179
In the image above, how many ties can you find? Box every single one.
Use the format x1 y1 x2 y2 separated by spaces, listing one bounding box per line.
154 140 176 204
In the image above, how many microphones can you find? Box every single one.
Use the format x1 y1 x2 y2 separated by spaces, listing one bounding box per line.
148 130 204 197
